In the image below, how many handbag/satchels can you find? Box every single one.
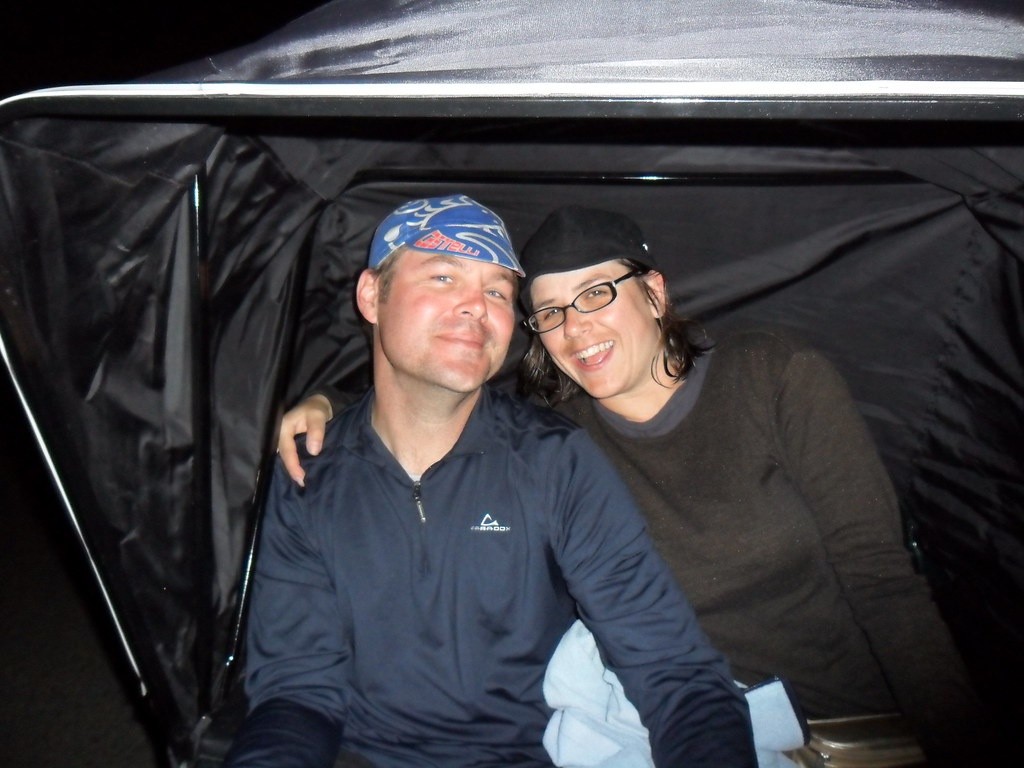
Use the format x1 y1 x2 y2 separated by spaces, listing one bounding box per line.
782 712 926 768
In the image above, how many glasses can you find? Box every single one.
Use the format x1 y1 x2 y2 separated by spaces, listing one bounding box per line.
523 269 639 334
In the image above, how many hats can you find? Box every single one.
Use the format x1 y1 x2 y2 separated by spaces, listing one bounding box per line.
519 204 657 315
368 194 526 268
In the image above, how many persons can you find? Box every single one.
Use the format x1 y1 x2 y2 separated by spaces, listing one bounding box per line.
224 194 1024 768
